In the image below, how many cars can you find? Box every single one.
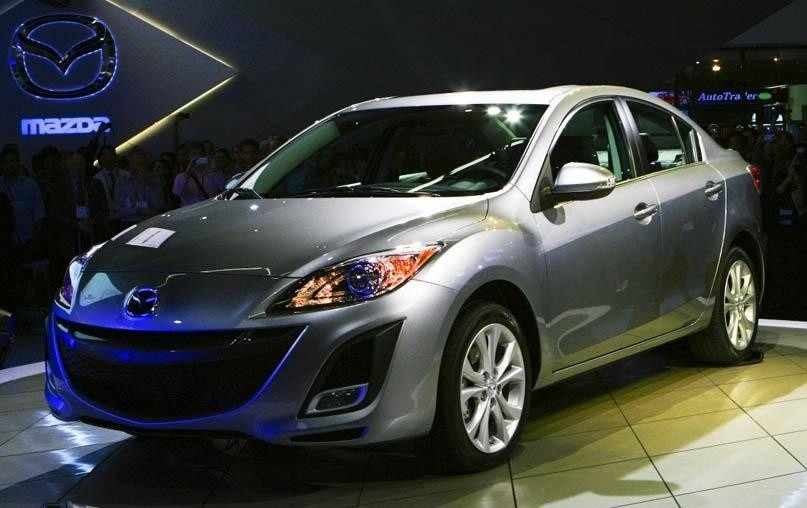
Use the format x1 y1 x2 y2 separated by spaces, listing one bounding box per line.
44 84 767 473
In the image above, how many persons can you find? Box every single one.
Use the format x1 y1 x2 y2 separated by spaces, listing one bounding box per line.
1 134 277 343
702 107 807 263
